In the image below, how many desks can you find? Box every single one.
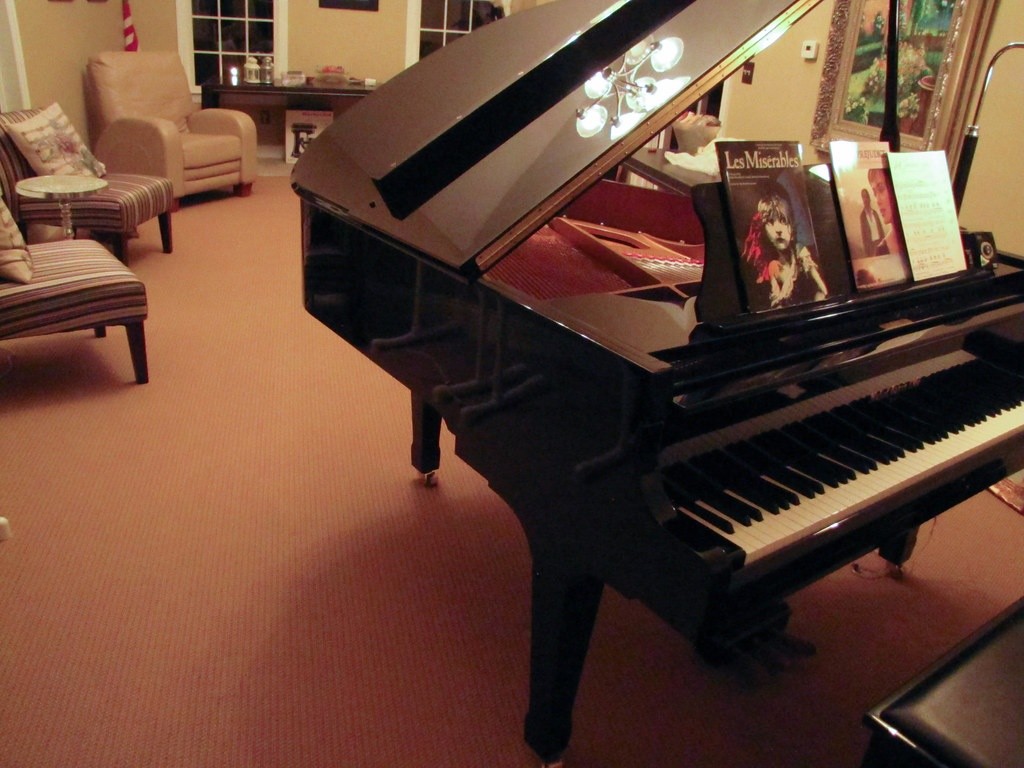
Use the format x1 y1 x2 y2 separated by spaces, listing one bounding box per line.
200 74 383 110
615 146 727 198
16 174 108 240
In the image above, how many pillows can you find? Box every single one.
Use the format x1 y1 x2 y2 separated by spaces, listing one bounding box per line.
6 101 106 179
1 198 36 284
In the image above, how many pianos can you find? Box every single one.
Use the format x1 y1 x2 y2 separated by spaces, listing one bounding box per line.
289 0 1024 768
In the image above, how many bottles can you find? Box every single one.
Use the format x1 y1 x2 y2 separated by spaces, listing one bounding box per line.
260 56 274 84
244 57 260 83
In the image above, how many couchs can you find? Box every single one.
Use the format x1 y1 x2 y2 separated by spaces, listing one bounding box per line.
81 50 258 212
0 109 176 267
0 243 151 385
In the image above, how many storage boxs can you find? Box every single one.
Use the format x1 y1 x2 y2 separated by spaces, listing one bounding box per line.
284 104 333 165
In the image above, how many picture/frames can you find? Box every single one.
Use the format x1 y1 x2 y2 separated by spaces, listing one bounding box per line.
810 0 996 160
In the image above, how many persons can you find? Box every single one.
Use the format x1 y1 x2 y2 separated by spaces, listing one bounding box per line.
859 188 890 257
868 169 906 252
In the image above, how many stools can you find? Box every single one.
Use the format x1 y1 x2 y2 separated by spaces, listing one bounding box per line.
859 593 1024 767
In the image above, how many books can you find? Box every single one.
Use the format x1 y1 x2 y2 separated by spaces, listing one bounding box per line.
716 141 828 315
829 141 912 292
